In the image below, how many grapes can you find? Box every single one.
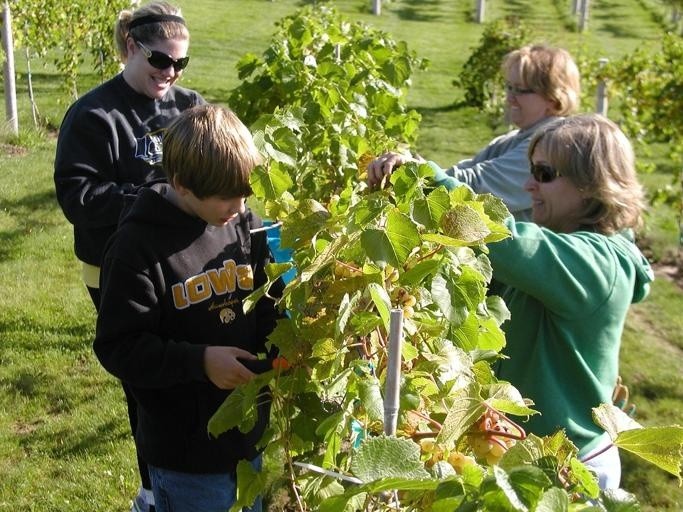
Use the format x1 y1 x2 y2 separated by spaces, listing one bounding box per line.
465 411 517 466
335 260 360 277
389 286 416 318
421 438 466 476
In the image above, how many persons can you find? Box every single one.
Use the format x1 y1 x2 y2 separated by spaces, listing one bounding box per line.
413 45 581 225
94 105 291 512
54 2 207 512
367 113 653 507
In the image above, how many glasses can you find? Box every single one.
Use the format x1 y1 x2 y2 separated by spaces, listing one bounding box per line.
509 86 536 95
531 161 560 182
134 38 190 72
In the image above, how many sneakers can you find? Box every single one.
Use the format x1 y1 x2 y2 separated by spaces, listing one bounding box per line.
132 487 154 511
